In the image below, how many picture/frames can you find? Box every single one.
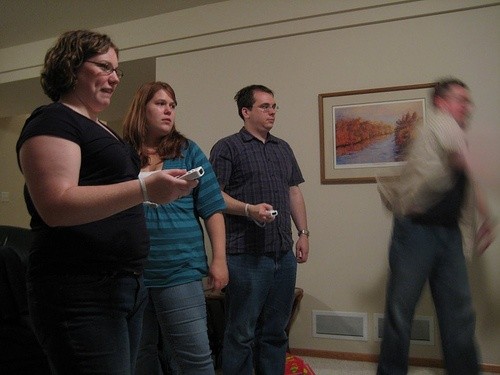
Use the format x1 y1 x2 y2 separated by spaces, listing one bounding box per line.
318 84 437 184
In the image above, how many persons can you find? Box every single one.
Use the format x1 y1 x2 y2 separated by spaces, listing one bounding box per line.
376 76 497 375
16 30 198 375
123 81 229 375
209 84 308 375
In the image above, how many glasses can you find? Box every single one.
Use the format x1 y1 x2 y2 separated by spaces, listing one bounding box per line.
85 60 124 79
256 105 279 112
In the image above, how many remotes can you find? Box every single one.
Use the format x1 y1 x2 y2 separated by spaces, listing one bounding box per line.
179 166 205 180
263 209 279 219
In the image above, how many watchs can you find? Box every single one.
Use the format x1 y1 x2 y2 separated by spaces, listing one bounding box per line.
298 230 309 238
